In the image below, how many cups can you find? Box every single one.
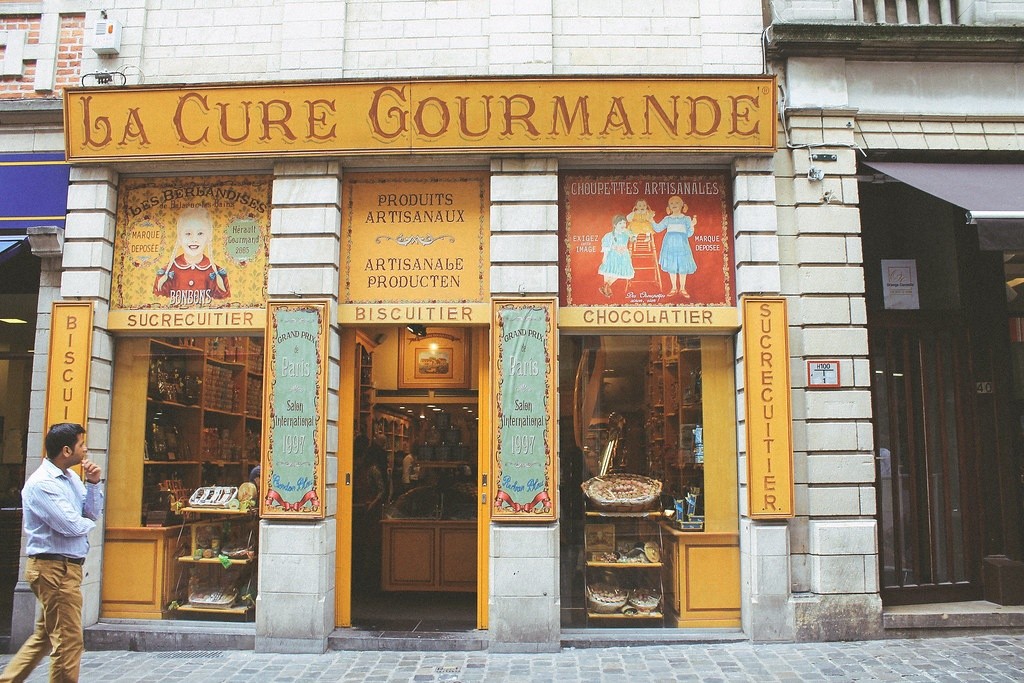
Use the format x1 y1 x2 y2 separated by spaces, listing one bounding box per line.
211 536 221 557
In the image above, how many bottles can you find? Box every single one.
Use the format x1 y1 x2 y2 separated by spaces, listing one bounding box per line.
415 464 420 474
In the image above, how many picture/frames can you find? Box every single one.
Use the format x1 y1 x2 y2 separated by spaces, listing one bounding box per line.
397 326 471 389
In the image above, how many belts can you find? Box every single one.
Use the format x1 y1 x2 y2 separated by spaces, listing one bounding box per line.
29 553 85 565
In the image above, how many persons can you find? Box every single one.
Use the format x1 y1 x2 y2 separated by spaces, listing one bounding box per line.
354 430 420 548
0 423 102 683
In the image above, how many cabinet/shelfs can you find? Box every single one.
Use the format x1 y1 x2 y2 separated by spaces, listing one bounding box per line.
100 327 416 619
582 336 741 629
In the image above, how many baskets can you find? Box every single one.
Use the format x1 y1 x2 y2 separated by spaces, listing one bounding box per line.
587 583 629 613
629 587 661 612
581 474 663 512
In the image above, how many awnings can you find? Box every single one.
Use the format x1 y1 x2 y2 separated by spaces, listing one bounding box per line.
866 159 1024 228
